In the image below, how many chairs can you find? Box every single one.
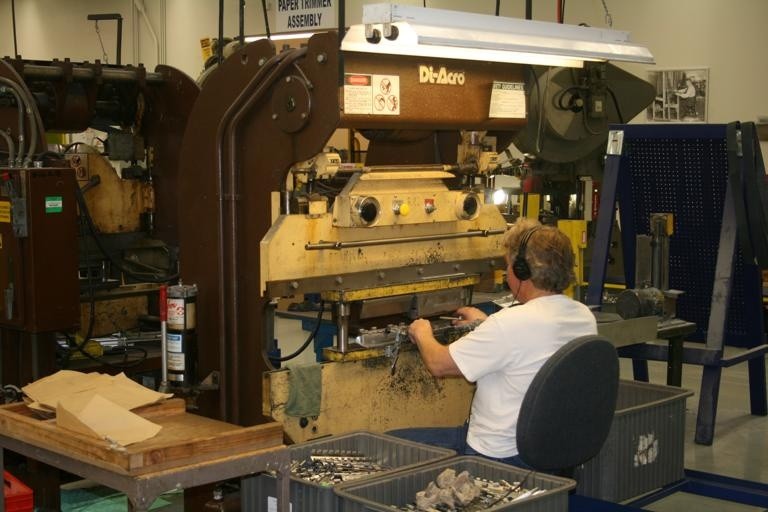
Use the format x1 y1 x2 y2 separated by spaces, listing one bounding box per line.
516 335 620 469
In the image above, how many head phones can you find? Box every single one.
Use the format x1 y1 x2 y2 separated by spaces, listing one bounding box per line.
513 224 541 280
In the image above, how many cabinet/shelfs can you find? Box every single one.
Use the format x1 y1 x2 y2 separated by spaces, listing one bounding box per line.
2 382 290 511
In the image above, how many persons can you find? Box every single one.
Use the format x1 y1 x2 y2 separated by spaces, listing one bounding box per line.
381 214 601 479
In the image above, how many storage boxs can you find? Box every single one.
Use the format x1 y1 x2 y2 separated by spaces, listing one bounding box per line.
569 380 694 506
240 429 456 510
332 456 575 511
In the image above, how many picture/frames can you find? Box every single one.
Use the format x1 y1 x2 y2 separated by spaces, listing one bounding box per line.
646 67 709 124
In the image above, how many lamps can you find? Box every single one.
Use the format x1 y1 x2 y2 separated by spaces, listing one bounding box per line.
340 0 655 68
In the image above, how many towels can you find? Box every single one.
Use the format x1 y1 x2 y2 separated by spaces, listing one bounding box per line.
283 365 322 418
